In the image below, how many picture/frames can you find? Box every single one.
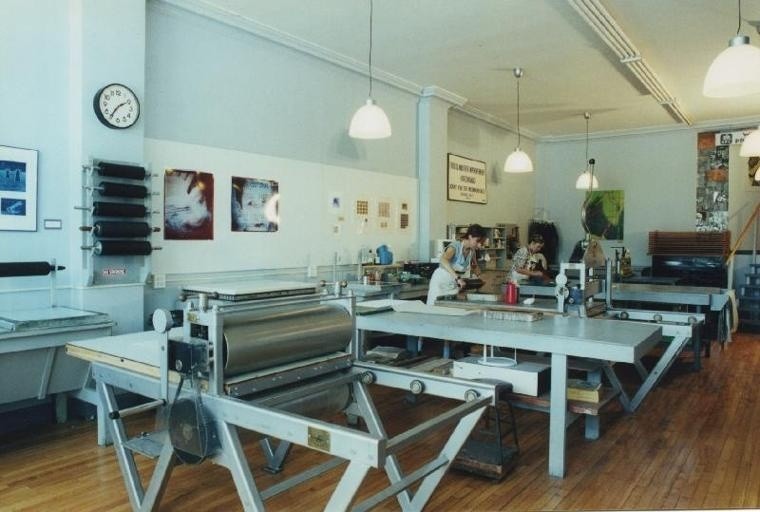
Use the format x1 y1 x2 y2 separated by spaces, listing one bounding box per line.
447 153 487 204
0 144 40 232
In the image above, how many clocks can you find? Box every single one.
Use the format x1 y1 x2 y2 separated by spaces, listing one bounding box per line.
93 82 140 130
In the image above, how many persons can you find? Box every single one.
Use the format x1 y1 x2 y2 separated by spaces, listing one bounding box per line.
506 234 544 296
417 224 486 356
165 171 211 233
236 180 274 232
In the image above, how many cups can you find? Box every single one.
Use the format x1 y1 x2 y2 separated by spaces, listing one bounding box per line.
507 284 517 305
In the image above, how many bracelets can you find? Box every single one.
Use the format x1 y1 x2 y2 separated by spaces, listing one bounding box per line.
455 276 461 281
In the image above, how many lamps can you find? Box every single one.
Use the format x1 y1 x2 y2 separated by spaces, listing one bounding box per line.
348 0 392 141
703 0 760 99
503 69 534 174
576 111 599 191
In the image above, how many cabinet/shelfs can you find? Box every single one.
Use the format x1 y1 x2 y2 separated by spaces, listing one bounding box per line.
446 224 508 271
